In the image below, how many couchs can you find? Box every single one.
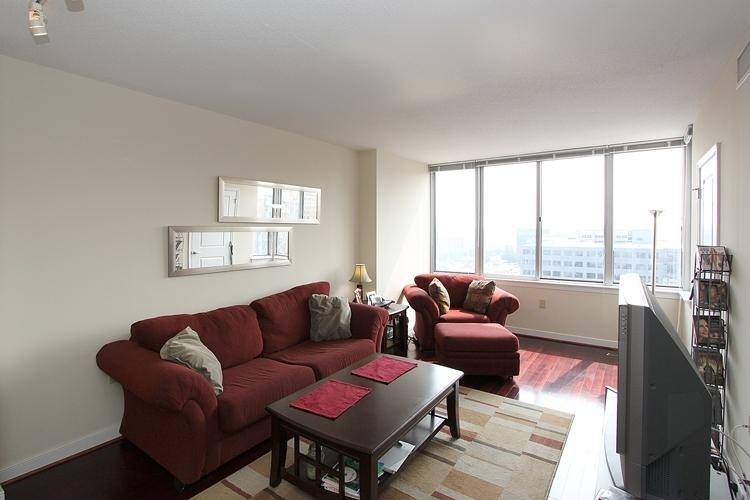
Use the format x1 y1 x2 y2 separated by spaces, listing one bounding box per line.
95 281 389 489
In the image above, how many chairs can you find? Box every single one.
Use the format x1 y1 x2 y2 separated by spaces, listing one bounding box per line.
402 273 520 353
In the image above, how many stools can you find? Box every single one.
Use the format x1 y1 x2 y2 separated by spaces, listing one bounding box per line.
433 321 520 381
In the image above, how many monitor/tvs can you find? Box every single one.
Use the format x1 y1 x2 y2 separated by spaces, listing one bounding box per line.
614 272 712 500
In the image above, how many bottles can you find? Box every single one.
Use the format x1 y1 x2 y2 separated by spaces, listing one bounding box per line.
305 443 325 481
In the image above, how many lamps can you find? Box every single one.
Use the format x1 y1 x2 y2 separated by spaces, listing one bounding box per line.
348 263 372 304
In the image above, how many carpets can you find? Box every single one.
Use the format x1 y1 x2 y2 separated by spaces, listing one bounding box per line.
185 383 575 499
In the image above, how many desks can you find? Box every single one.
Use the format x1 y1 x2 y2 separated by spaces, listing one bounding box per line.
352 299 409 358
594 385 734 500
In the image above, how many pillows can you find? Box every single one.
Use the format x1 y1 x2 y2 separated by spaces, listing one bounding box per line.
158 324 224 399
428 277 451 315
308 293 353 343
463 279 496 314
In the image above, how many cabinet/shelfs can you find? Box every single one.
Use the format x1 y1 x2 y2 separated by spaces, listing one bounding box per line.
690 245 734 471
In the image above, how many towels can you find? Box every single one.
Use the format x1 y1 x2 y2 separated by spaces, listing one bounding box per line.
288 355 418 419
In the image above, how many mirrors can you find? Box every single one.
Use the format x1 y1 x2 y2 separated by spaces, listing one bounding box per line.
168 175 321 278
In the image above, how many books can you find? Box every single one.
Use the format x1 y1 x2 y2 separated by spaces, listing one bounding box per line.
692 245 731 388
